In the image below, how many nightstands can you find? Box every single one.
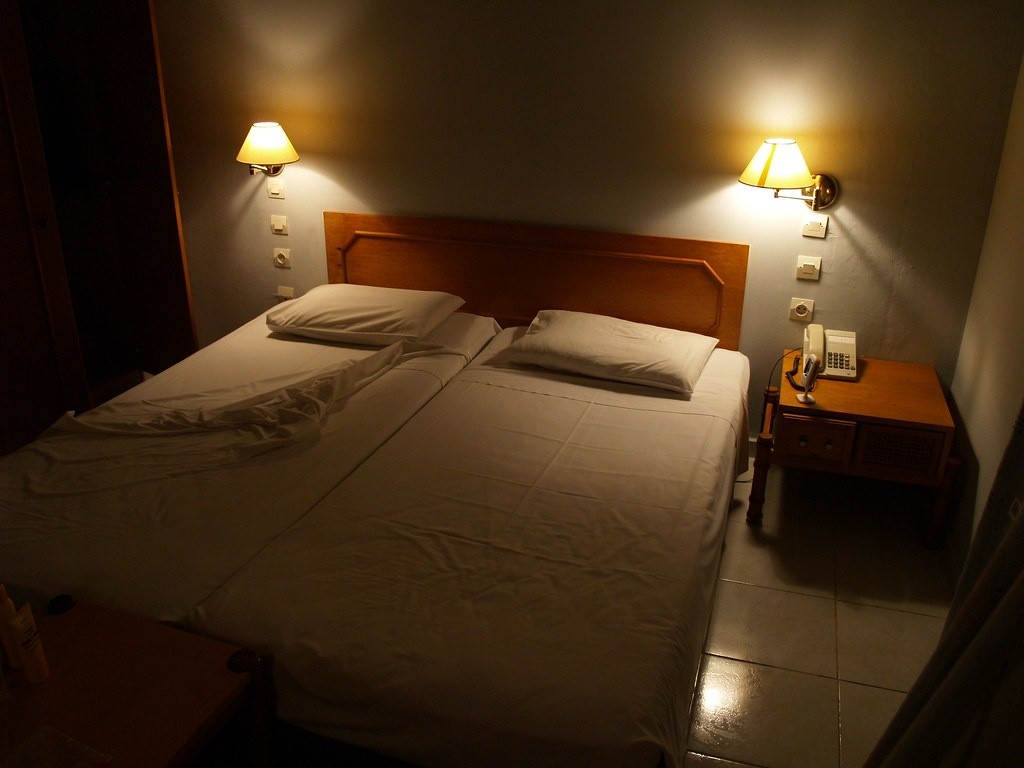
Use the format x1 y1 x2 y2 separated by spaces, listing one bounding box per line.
745 350 955 551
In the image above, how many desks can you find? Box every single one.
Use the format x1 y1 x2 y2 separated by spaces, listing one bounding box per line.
0 596 268 768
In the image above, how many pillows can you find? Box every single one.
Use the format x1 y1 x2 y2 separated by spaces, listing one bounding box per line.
500 309 720 393
266 283 466 346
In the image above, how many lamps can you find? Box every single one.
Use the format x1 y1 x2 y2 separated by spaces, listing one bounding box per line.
738 139 839 211
235 121 300 175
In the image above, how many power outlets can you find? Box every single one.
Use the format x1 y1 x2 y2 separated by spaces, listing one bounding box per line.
273 247 291 268
789 297 815 322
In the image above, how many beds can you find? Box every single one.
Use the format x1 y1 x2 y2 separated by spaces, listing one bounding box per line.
1 211 752 768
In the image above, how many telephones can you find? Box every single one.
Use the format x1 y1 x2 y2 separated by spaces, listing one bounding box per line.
801 323 859 383
